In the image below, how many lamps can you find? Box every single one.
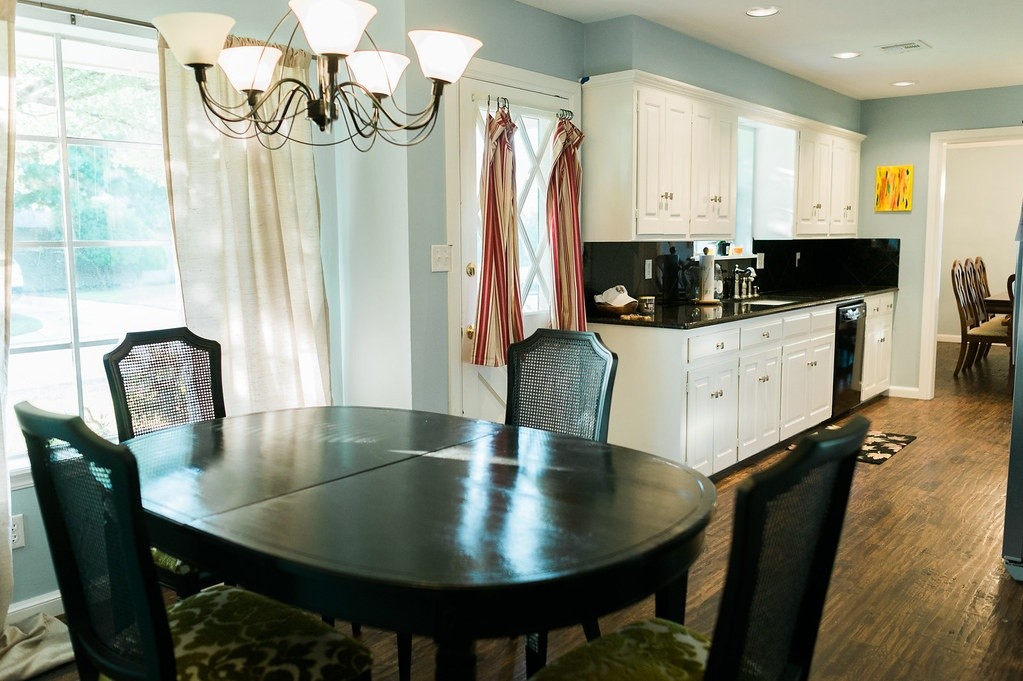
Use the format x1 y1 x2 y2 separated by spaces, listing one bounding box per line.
151 1 485 152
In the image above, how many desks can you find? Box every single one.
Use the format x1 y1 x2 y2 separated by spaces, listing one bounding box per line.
984 291 1014 314
87 406 718 679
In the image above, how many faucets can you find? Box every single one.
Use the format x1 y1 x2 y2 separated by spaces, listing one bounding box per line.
734 264 757 300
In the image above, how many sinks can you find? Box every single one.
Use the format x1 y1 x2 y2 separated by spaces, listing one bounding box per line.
743 300 800 306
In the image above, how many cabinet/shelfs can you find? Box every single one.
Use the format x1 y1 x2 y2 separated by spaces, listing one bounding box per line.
860 291 894 406
751 103 868 241
586 311 783 484
780 301 837 450
579 68 738 243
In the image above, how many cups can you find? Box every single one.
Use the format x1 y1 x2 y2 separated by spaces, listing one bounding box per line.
639 297 655 315
730 241 743 256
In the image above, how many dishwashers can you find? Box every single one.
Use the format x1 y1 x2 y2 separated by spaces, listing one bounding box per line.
831 299 867 420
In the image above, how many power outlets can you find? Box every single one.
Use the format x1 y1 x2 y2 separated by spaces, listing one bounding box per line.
11 513 25 549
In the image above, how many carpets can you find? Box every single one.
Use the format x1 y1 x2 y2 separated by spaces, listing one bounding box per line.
784 423 918 466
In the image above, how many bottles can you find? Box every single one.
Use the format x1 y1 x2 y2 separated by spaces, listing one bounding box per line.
714 264 724 299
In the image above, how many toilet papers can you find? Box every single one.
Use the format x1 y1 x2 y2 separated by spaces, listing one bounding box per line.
699 254 715 300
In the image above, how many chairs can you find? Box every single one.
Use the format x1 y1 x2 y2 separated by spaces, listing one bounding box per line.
525 415 872 681
12 399 375 681
396 328 619 679
951 255 1016 400
101 326 363 639
